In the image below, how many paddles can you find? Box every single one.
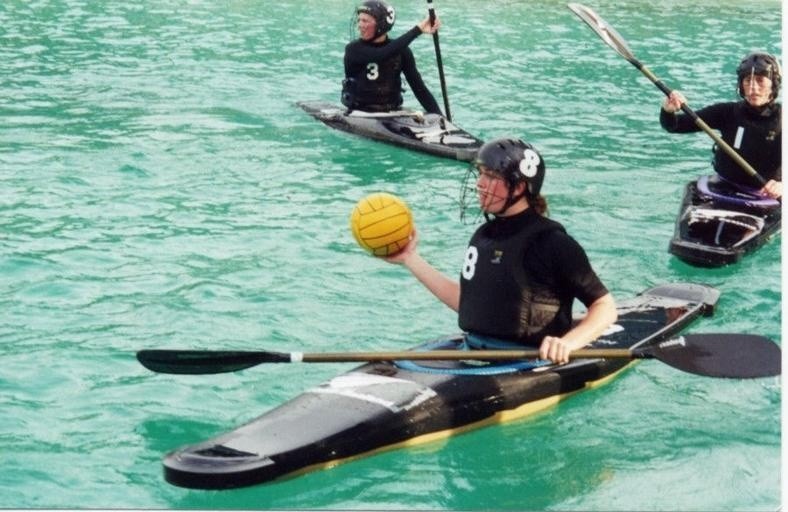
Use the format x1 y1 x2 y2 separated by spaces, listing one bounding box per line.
568 2 781 204
136 334 781 378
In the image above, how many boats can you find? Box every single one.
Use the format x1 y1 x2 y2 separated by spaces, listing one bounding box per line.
664 175 782 271
158 280 724 494
298 93 485 165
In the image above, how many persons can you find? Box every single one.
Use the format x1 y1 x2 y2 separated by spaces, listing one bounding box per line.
338 1 444 116
374 133 623 368
657 49 782 202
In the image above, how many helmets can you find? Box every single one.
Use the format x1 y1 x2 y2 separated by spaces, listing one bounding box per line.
474 138 545 197
357 0 396 40
736 53 782 108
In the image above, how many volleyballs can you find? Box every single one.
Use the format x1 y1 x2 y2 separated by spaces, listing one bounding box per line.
351 192 413 257
